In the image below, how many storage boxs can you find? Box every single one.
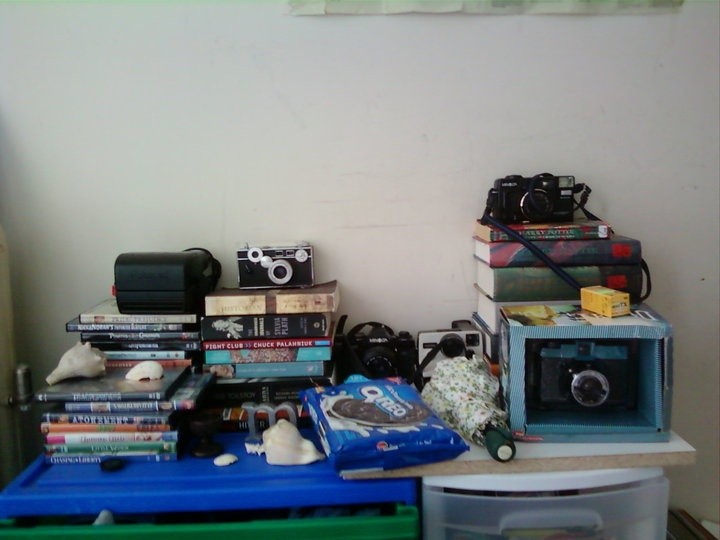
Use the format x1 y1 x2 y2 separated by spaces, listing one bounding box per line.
0 426 423 540
420 466 669 540
498 300 675 443
580 285 629 318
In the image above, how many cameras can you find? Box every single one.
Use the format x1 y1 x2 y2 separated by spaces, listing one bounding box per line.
416 319 483 385
491 175 575 226
538 340 631 407
237 241 314 289
335 330 418 384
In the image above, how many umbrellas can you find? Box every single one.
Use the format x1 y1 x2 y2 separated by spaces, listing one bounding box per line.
420 356 516 463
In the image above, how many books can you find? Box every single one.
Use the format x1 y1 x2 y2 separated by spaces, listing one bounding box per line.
35 280 341 464
472 315 499 364
472 235 641 267
473 283 581 338
473 258 643 301
475 217 611 243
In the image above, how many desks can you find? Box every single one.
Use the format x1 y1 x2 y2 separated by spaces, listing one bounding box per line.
340 427 699 480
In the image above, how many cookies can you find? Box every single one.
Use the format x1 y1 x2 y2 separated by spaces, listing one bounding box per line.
326 399 431 427
